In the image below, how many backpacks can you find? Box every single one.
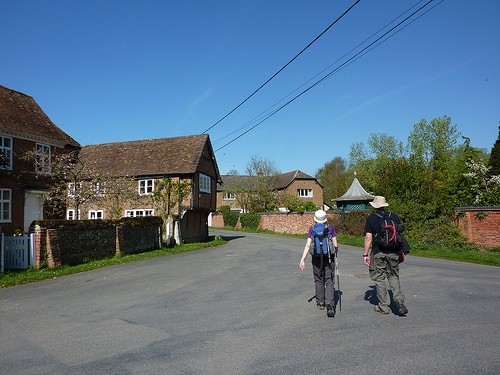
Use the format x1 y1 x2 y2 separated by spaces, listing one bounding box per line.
311 224 335 255
373 212 400 247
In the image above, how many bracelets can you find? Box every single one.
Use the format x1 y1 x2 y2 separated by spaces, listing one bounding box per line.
364 255 369 257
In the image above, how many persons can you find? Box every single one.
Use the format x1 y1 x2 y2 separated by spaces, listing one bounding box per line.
364 196 408 315
300 210 339 317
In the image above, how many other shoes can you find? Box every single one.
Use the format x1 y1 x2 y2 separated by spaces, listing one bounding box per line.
317 302 325 309
374 305 389 314
395 299 408 315
326 302 335 317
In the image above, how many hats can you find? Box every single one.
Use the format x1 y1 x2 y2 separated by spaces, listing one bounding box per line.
314 210 327 224
369 196 389 208
